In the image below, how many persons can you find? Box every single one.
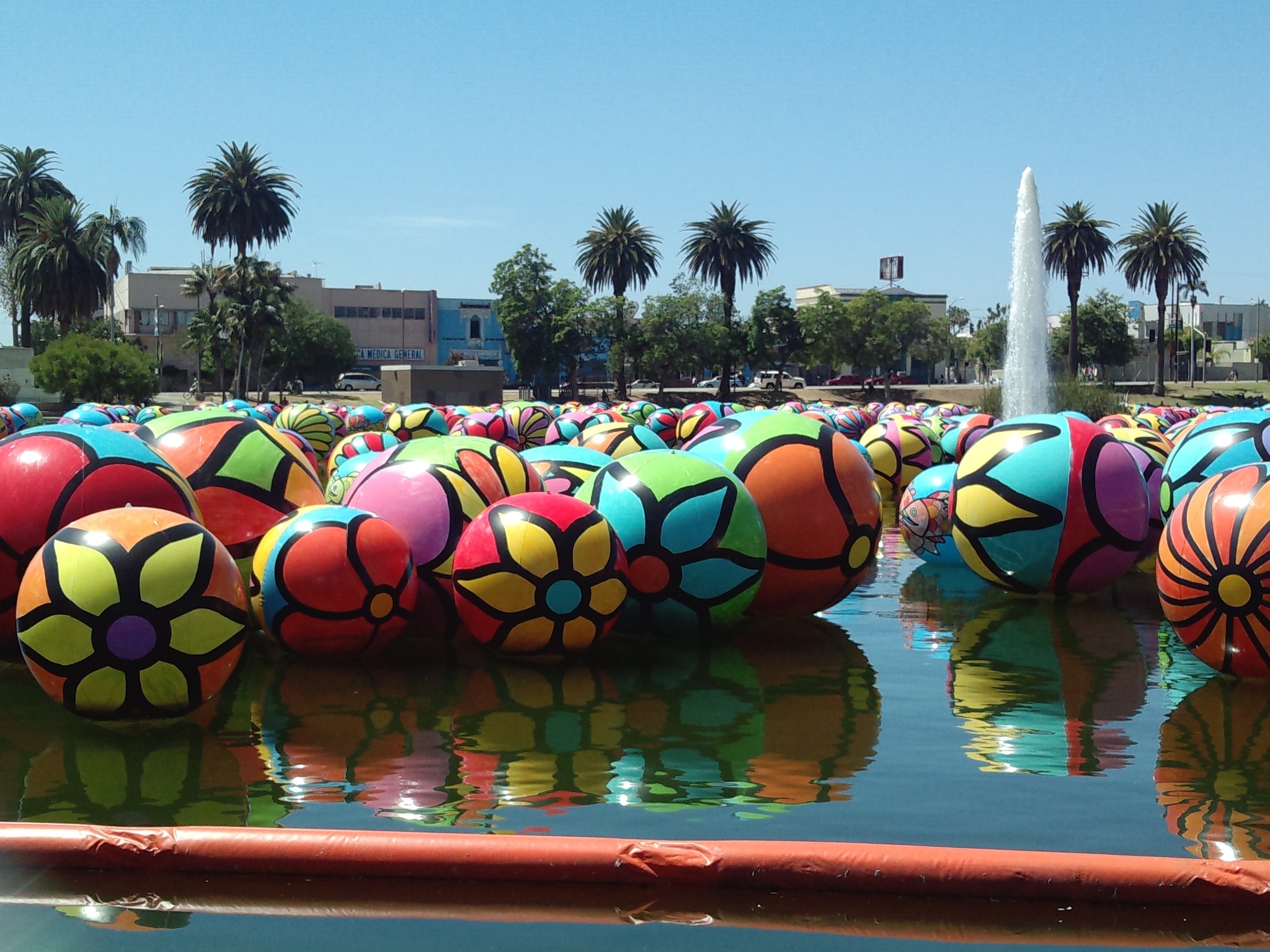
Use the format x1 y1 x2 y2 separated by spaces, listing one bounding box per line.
680 371 698 387
319 375 330 395
190 377 200 397
1229 368 1235 382
1233 369 1239 383
1080 363 1098 384
776 371 784 392
284 374 305 395
958 373 962 383
601 390 610 402
940 374 944 384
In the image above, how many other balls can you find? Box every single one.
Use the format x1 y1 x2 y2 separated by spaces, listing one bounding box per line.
0 397 1270 719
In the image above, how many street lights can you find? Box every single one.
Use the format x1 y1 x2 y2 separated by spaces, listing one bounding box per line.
1164 317 1208 382
946 296 974 384
